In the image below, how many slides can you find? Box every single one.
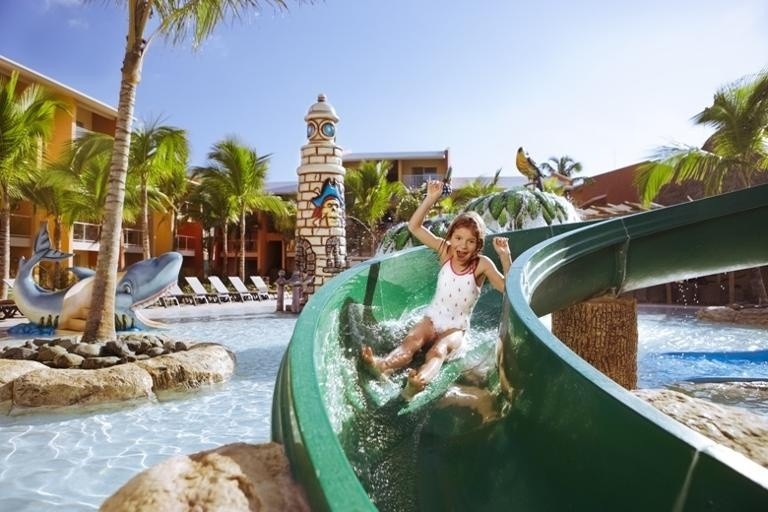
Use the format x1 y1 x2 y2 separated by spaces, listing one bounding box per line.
272 181 768 510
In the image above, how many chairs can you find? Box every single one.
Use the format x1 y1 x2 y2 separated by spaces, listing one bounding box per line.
160 275 272 308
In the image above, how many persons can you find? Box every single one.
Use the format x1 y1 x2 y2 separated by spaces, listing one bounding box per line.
359 174 515 404
